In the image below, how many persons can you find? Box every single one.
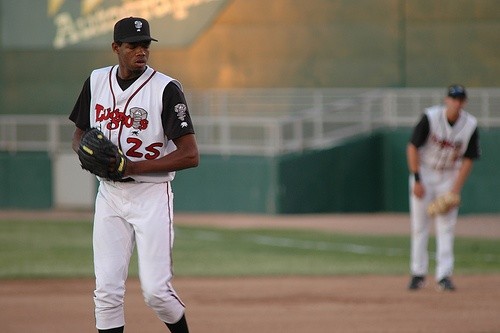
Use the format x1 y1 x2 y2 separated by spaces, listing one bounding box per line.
68 16 200 333
407 84 480 290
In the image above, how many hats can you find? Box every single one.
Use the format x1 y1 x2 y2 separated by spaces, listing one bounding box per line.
113 16 158 42
448 84 468 100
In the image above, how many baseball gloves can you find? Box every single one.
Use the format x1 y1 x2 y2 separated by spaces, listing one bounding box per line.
426 191 460 217
76 128 136 183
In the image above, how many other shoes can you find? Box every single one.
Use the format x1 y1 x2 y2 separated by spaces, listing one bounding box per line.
410 274 424 287
438 277 456 292
